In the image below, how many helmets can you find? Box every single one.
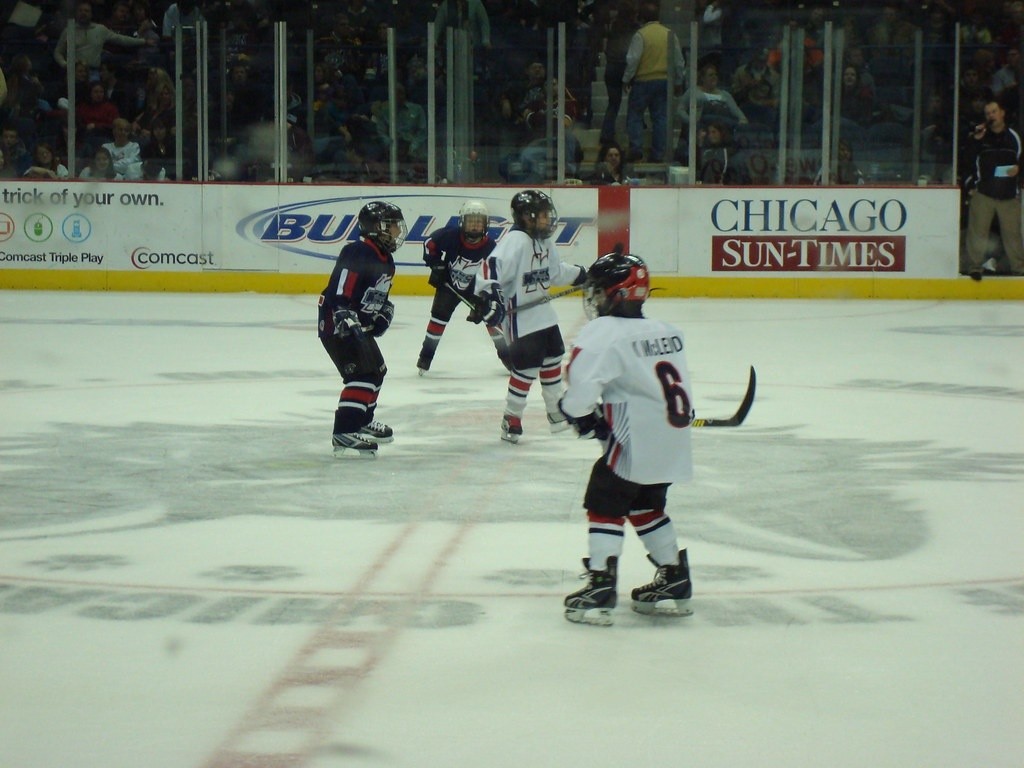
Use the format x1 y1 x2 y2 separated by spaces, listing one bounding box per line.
357 201 408 253
458 201 490 239
510 189 559 240
583 252 649 320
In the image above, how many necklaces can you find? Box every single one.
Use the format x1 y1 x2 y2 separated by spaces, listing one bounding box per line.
159 144 165 154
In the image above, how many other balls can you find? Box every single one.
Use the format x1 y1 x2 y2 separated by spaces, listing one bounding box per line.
971 272 981 281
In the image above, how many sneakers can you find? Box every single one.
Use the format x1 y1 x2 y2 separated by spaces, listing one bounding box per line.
500 412 523 445
358 403 394 442
563 555 618 626
332 409 378 458
547 411 570 433
416 349 434 375
631 548 692 614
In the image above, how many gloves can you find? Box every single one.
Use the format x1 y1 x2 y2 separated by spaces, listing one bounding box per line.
333 309 366 345
367 299 394 338
429 264 453 292
557 398 610 441
467 294 490 325
484 282 506 326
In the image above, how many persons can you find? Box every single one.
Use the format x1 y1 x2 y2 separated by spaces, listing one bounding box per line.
317 201 409 450
558 252 695 610
967 99 1024 281
473 189 588 435
416 198 514 371
0 0 1024 187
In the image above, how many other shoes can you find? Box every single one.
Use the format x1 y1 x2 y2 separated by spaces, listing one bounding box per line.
970 273 981 280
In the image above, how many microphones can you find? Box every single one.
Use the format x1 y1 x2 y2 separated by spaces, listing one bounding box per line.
976 119 994 134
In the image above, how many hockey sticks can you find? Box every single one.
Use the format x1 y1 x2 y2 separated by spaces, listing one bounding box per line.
502 241 625 317
444 281 504 336
692 365 757 427
338 303 374 339
